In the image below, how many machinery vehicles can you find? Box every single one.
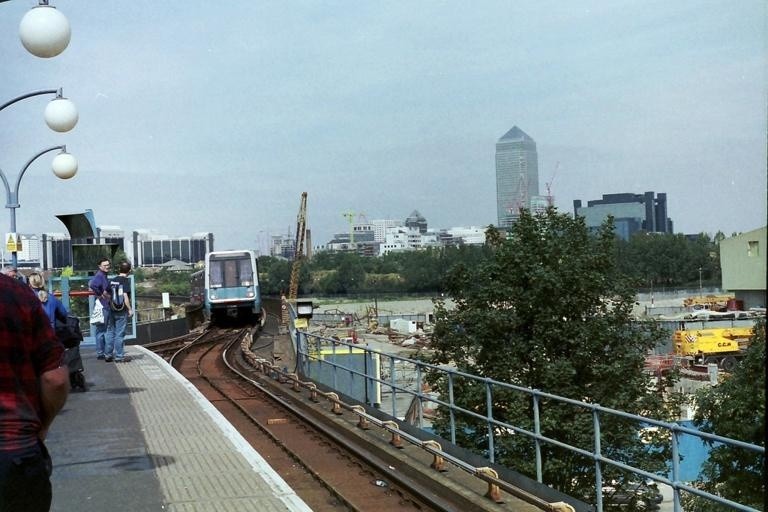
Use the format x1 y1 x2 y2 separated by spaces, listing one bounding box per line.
283 191 319 332
683 292 733 311
673 323 768 374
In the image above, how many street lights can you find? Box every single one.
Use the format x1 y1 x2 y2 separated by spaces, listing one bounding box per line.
1 1 78 283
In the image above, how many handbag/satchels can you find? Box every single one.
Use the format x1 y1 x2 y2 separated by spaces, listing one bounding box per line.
91 299 104 325
56 316 82 347
109 284 125 313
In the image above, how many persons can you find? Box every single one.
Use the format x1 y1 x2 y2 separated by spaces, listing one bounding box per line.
0 265 18 280
99 261 135 363
0 271 68 510
89 257 115 362
27 272 67 331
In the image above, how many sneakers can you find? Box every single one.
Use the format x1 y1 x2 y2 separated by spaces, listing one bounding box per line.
98 355 130 362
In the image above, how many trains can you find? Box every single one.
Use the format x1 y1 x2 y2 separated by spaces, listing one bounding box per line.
186 247 262 332
